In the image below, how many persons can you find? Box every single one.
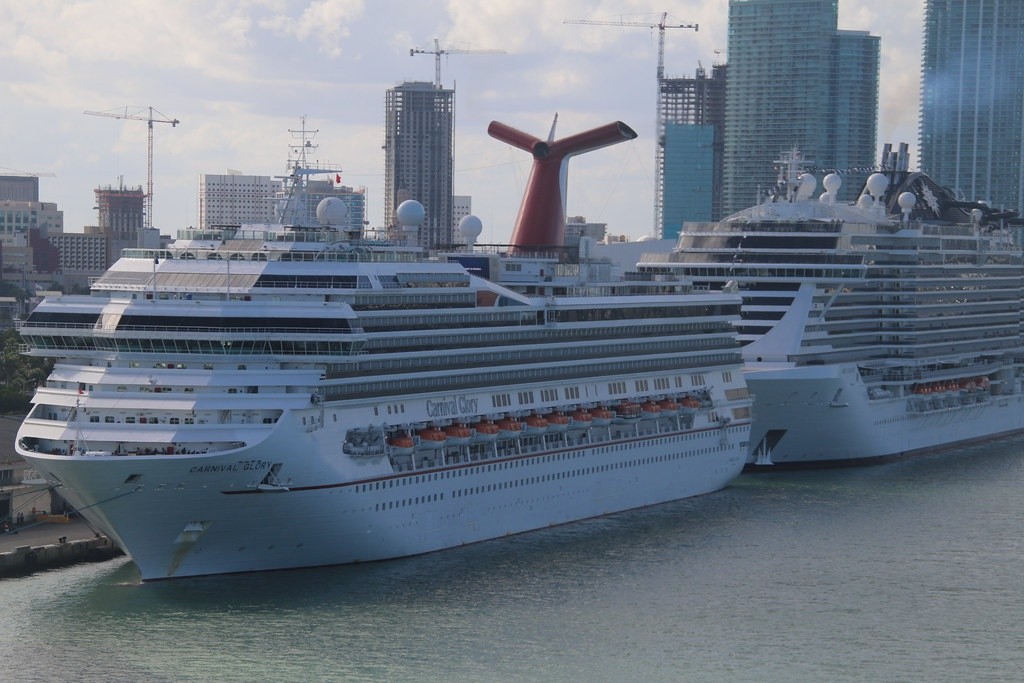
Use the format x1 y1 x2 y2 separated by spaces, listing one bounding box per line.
17 512 24 524
51 448 66 455
112 447 207 456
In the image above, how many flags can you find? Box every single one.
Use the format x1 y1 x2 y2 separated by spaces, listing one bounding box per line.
78 384 84 394
336 174 341 183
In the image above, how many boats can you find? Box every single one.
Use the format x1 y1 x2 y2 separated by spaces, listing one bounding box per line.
635 142 1024 464
15 111 757 583
33 511 69 523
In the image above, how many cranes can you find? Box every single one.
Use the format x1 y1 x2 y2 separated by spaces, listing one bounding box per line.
83 105 181 228
409 39 507 87
563 12 699 241
